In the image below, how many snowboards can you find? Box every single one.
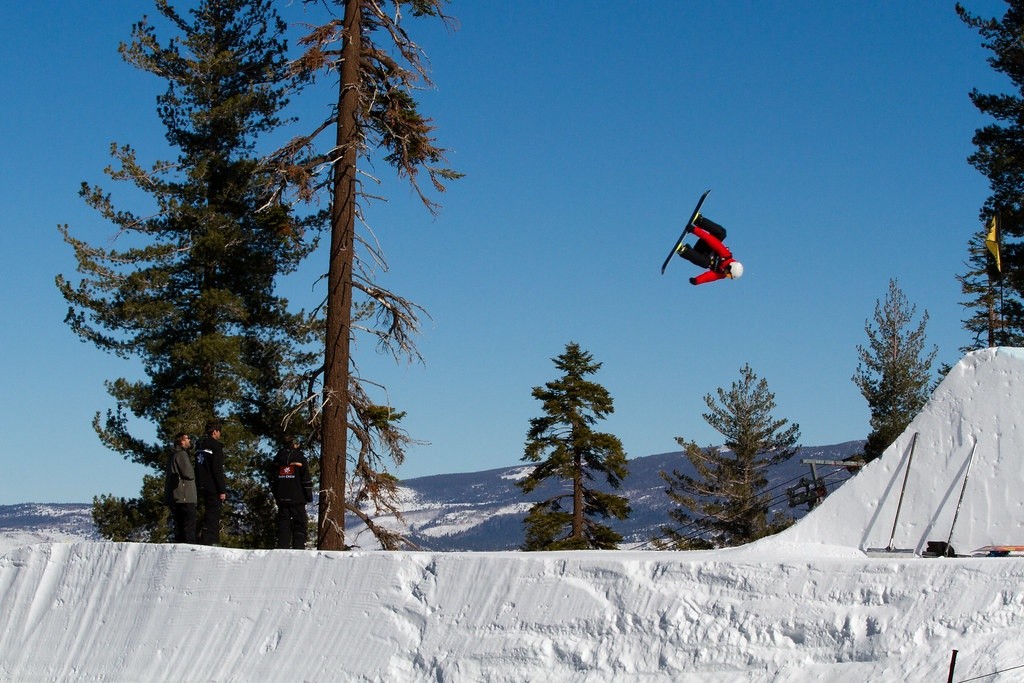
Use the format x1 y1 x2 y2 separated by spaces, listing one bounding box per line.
657 182 713 277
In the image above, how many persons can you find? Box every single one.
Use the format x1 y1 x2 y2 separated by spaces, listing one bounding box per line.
269 433 313 550
675 212 743 285
164 432 197 545
194 421 226 547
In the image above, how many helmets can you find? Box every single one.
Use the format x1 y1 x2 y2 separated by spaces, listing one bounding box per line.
729 262 743 279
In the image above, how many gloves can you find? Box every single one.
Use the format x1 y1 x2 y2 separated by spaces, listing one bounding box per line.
685 225 694 233
689 278 696 285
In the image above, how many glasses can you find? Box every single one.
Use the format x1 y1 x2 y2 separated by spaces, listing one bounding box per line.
294 443 298 445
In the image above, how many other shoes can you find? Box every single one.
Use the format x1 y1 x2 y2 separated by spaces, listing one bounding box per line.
675 243 687 254
692 212 702 225
